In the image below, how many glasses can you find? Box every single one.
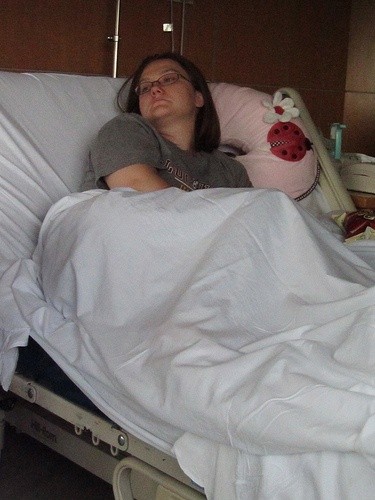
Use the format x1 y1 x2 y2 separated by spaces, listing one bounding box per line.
135 73 192 95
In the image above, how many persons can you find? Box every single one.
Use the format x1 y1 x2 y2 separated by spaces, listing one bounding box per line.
89 53 256 196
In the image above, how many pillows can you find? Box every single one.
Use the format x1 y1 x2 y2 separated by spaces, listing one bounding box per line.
203 81 321 206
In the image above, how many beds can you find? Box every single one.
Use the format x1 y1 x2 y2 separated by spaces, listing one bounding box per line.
0 66 375 500
